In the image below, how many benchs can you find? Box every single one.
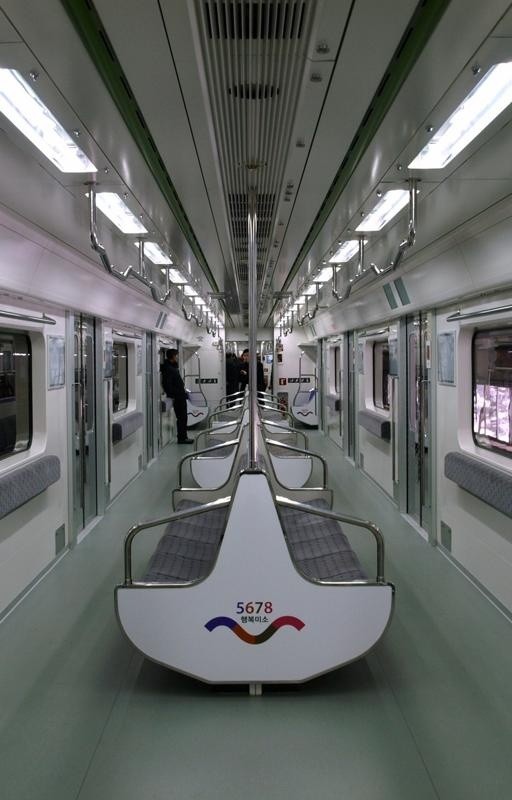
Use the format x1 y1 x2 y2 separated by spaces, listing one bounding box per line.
115 390 395 699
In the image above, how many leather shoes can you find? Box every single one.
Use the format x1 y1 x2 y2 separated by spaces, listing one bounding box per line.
178 438 193 444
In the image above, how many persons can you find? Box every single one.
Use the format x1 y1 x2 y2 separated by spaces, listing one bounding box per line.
160 348 196 446
225 348 266 411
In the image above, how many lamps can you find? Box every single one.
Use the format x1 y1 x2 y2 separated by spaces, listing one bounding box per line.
273 265 345 327
72 185 154 236
400 65 507 178
0 67 100 182
130 239 223 329
322 232 370 267
349 182 421 239
